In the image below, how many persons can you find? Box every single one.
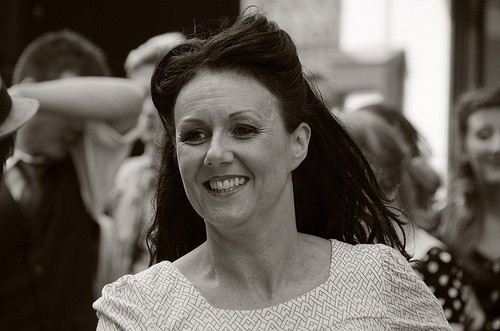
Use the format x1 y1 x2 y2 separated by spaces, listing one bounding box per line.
327 109 484 331
357 104 441 234
430 85 500 331
92 14 451 331
0 31 147 331
109 32 186 284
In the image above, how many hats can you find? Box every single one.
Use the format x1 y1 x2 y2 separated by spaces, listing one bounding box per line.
0 79 40 136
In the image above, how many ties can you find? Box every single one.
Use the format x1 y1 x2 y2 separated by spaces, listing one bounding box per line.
17 160 44 222
